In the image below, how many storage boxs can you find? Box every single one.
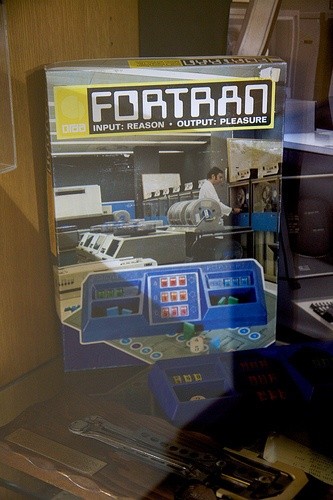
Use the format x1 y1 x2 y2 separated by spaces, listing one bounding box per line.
44 56 287 373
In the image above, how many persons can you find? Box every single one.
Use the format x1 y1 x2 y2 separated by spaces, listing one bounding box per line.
198 167 242 226
